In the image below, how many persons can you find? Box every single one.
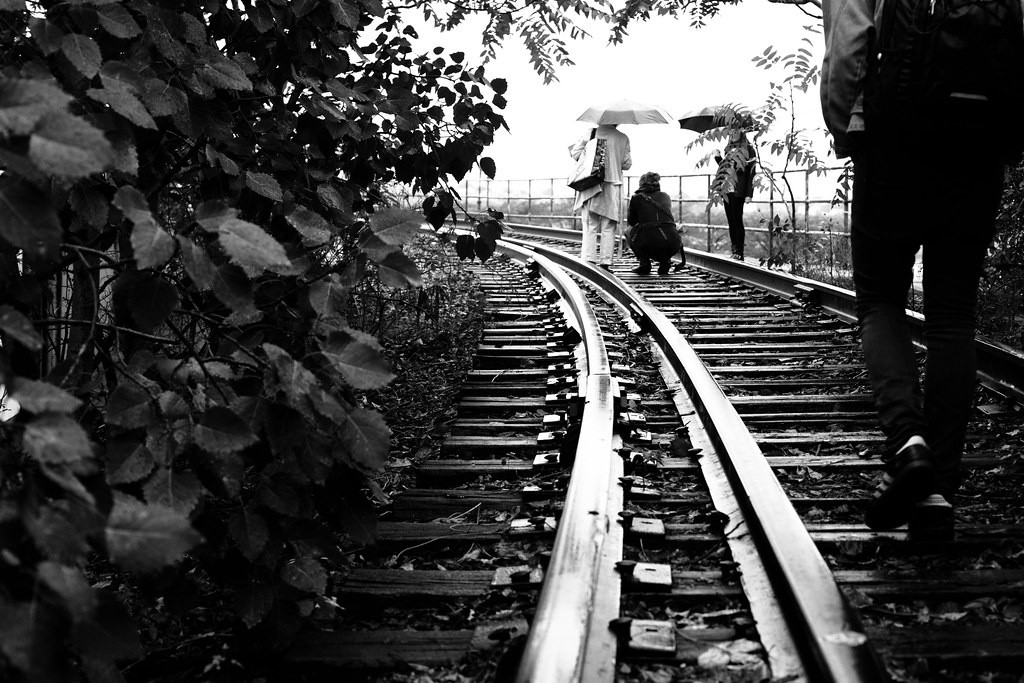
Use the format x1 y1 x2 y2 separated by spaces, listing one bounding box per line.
573 121 632 272
623 171 682 275
821 0 1024 546
713 123 758 262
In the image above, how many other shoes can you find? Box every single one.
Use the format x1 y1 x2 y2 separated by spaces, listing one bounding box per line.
631 261 651 273
730 254 744 261
657 261 671 274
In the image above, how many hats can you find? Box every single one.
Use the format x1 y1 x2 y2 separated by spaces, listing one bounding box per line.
639 172 661 187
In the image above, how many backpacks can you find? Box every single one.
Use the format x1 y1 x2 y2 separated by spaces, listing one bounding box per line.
861 0 1024 179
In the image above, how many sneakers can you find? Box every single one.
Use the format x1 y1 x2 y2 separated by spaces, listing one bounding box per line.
865 435 955 544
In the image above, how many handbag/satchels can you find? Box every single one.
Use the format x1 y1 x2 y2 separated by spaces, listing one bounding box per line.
568 128 607 193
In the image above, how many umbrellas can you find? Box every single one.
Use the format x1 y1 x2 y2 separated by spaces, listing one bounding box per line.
575 104 672 125
678 106 757 134
